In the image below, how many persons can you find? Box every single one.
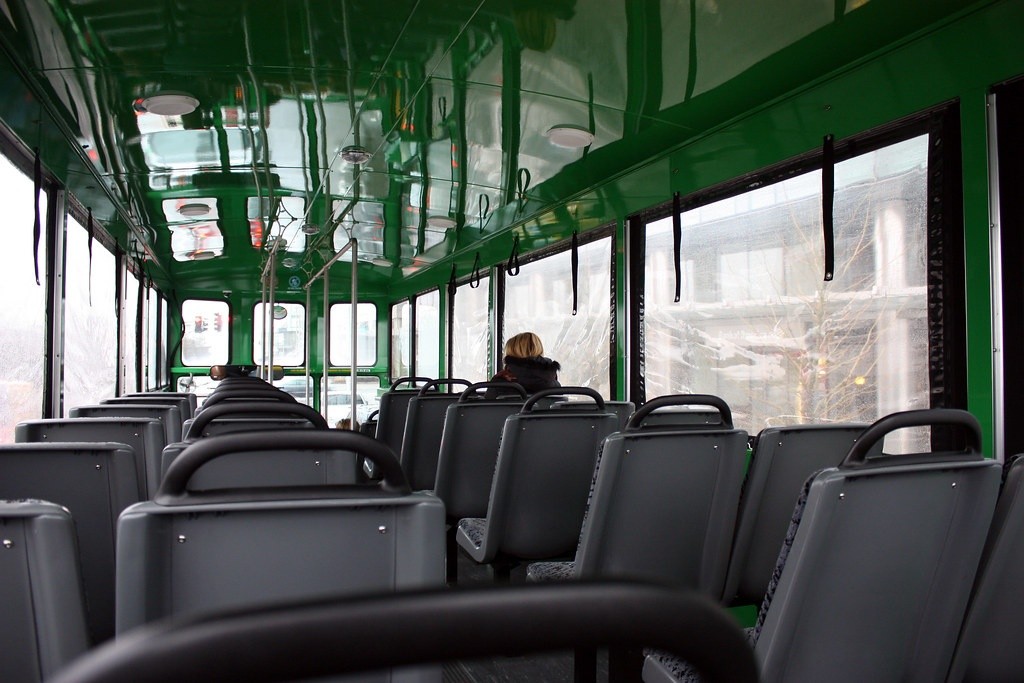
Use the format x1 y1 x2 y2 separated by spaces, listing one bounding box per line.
211 367 223 379
484 332 562 399
337 418 360 432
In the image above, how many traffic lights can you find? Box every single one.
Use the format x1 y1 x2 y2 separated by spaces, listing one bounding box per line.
195 316 203 333
202 317 208 332
214 312 222 332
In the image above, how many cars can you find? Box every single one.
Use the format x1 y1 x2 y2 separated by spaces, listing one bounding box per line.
271 375 369 430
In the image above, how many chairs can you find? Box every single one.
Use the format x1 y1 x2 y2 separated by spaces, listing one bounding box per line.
455 387 620 589
182 401 329 444
399 379 479 490
550 399 636 435
498 394 572 413
633 408 723 427
123 392 197 419
413 381 528 525
15 417 166 502
111 432 447 639
44 576 766 683
643 407 1003 683
948 450 1024 683
525 393 748 606
193 388 299 418
69 404 181 445
361 410 379 440
359 376 440 482
718 424 886 621
0 496 93 683
0 442 140 648
202 384 282 406
99 396 191 424
216 380 271 388
222 376 261 380
